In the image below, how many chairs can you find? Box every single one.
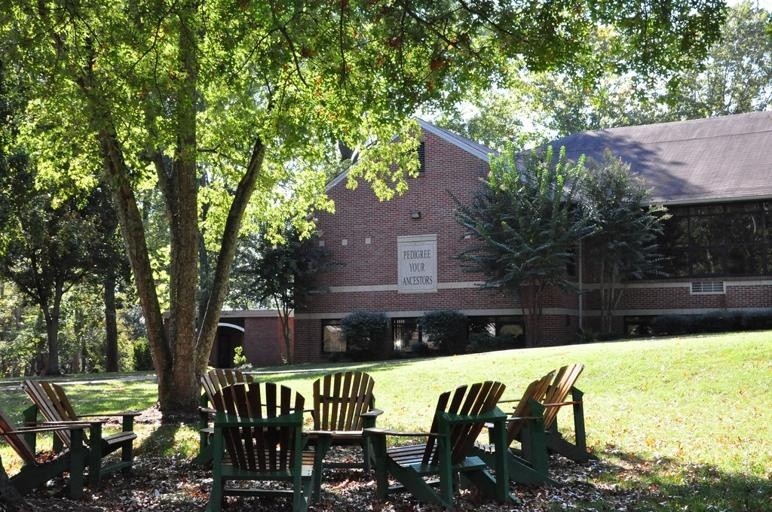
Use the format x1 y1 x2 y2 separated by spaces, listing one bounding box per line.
303 371 384 474
364 380 519 512
0 407 91 505
189 368 281 468
485 361 599 488
20 379 142 487
199 381 335 512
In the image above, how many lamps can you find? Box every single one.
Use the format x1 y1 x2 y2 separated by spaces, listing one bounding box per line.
411 210 421 219
